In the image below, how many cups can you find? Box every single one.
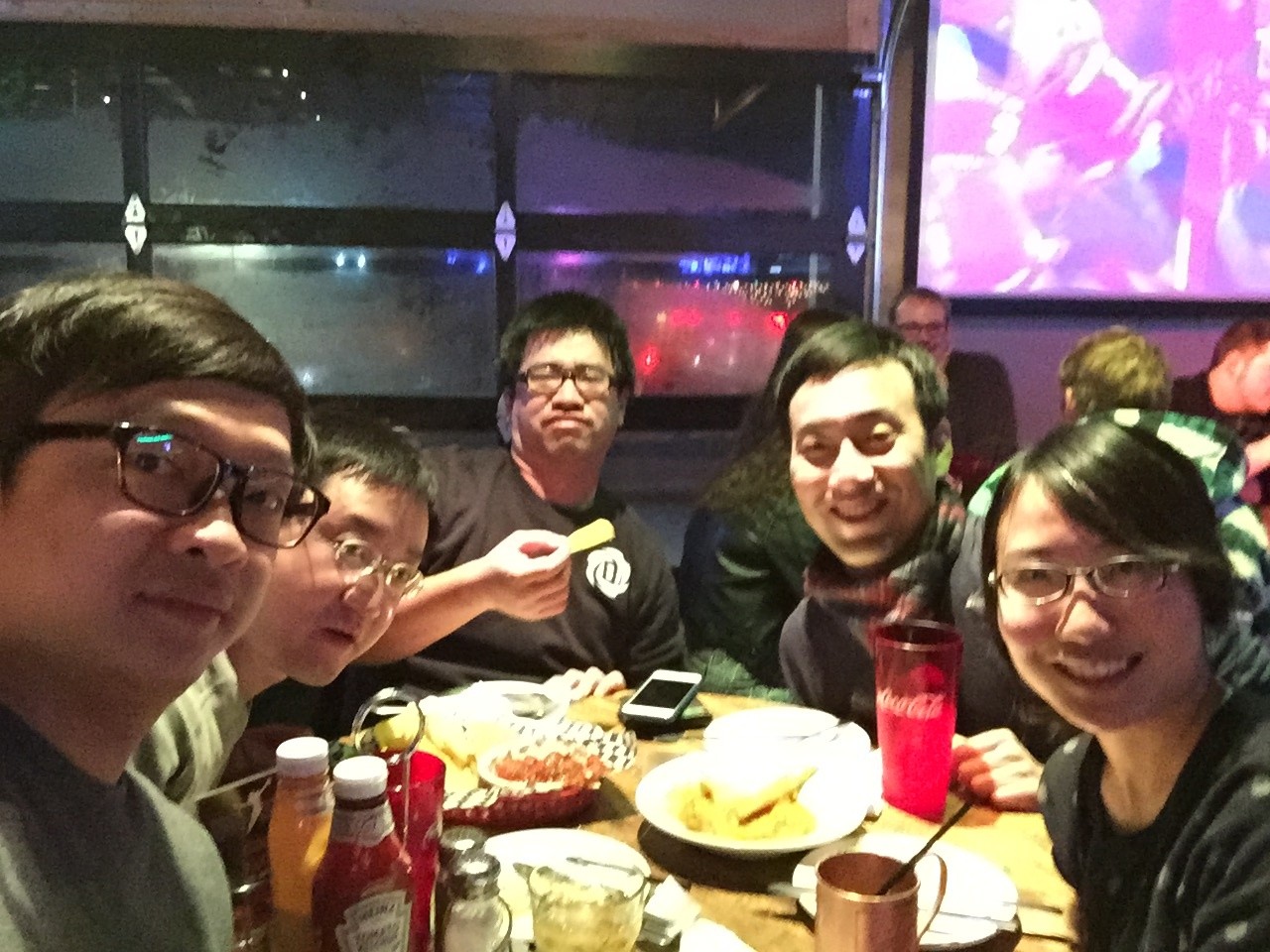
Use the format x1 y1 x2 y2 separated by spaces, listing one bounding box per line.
814 848 947 951
528 859 647 952
873 614 961 819
379 745 446 952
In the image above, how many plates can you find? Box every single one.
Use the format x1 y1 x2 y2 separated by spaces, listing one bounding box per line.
792 832 1017 949
702 706 871 756
478 825 650 944
634 742 871 856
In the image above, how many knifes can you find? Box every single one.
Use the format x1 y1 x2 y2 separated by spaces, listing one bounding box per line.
511 861 683 952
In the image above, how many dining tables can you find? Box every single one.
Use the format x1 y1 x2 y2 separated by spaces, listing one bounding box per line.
272 680 1090 951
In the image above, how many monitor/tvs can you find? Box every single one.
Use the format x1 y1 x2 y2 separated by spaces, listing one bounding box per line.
905 0 1270 318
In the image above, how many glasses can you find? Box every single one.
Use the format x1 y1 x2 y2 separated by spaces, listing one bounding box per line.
310 528 425 600
895 320 948 334
987 554 1191 606
22 420 331 550
514 362 624 398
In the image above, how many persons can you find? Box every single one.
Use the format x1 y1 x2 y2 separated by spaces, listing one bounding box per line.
0 272 328 947
980 420 1270 951
894 291 1021 514
1170 306 1270 516
777 322 1084 812
678 296 853 691
317 289 688 749
944 322 1270 631
127 402 437 814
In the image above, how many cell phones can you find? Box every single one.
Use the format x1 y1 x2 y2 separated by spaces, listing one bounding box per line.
621 669 703 725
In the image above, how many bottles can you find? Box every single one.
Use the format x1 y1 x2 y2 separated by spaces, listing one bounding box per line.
437 824 487 934
265 737 334 952
437 853 515 952
312 755 415 952
